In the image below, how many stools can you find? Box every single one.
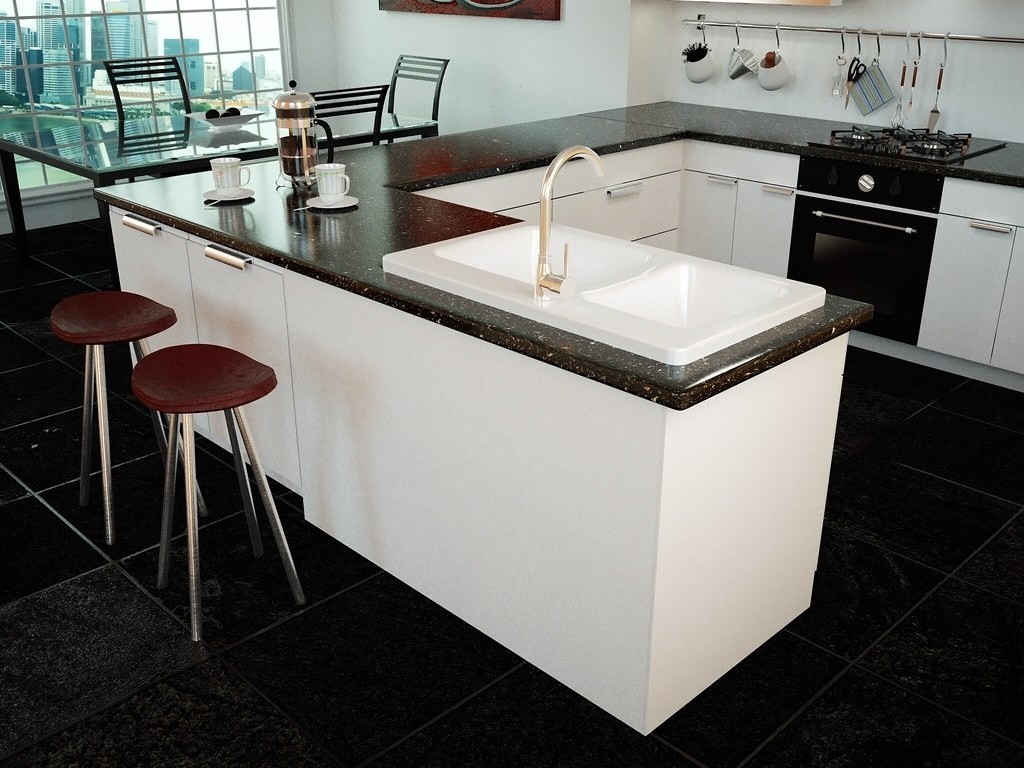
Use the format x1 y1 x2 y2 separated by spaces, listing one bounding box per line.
51 291 210 545
130 343 306 642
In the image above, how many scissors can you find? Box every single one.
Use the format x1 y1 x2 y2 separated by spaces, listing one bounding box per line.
845 57 867 110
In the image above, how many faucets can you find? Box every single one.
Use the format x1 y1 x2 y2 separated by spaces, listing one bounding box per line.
534 145 607 298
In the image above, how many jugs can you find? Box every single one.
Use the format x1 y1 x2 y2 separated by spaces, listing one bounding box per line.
270 80 334 188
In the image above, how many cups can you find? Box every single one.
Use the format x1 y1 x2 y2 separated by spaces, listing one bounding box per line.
757 55 790 91
210 157 251 198
314 163 350 203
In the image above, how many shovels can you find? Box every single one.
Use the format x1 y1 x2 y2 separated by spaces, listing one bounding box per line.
927 66 943 133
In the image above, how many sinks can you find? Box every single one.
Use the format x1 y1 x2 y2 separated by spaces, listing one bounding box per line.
381 218 678 318
534 252 826 366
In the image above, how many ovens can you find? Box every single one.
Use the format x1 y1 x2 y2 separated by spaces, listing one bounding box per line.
786 156 945 347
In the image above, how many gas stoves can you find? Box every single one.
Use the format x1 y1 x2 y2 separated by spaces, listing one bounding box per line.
796 125 1008 214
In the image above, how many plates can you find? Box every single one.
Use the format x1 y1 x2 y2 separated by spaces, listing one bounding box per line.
306 196 359 208
182 106 264 126
203 189 255 200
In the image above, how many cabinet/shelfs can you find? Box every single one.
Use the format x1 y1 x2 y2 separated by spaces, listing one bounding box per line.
412 138 801 280
916 175 1024 376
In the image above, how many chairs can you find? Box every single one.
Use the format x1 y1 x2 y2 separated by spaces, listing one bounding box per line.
387 55 450 144
310 84 391 153
102 55 191 182
116 117 189 157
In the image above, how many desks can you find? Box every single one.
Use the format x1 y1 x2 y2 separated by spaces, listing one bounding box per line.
0 105 439 290
